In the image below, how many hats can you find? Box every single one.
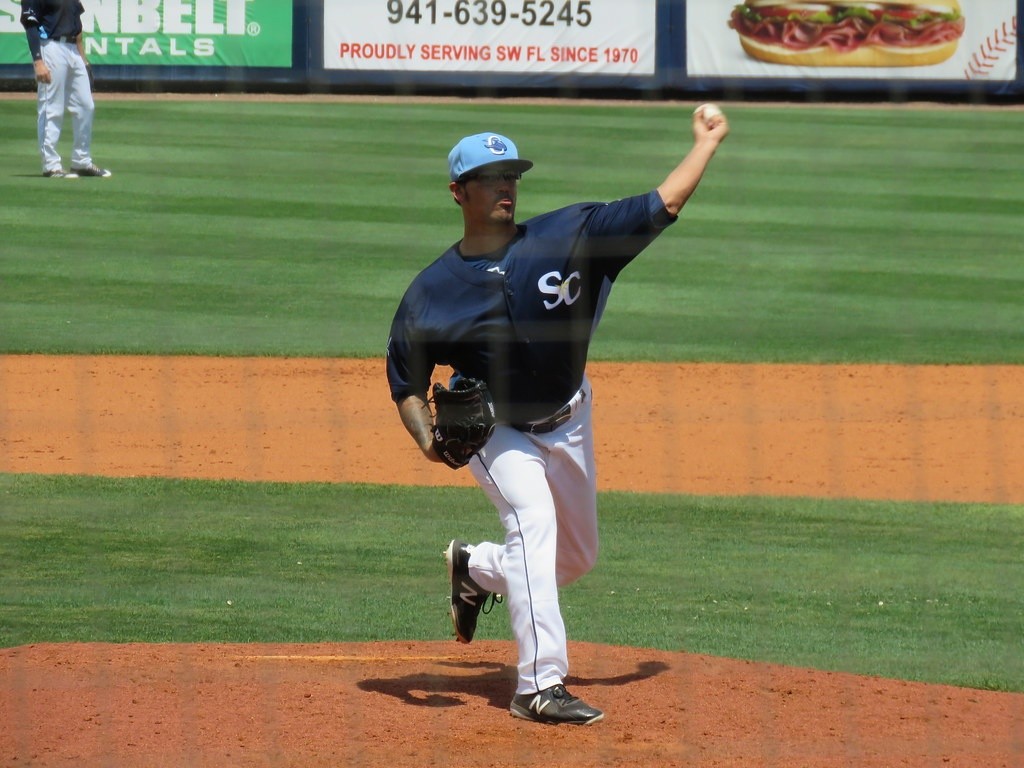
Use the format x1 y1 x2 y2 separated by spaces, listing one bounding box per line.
447 132 533 183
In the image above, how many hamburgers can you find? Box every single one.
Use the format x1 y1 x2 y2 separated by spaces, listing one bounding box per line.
728 0 964 67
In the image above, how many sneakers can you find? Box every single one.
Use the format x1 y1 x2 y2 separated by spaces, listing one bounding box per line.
70 164 110 177
443 539 503 644
510 685 604 725
43 169 78 178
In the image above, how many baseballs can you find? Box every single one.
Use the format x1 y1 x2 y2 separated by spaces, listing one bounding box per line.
695 102 724 126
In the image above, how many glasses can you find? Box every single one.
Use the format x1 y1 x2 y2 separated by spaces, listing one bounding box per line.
457 168 522 187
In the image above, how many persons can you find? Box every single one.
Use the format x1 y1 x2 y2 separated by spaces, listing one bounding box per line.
20 0 111 180
384 104 729 726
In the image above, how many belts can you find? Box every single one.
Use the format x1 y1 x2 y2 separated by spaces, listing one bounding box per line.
53 37 76 43
510 389 586 435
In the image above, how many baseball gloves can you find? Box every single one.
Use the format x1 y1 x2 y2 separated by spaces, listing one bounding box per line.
426 375 498 471
